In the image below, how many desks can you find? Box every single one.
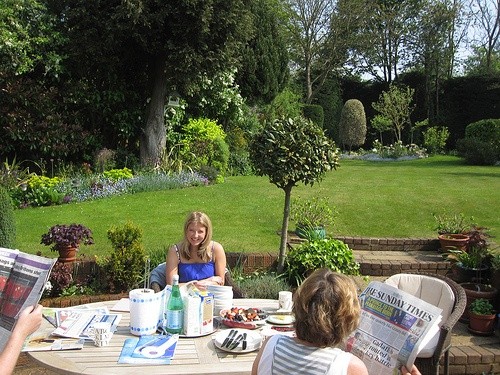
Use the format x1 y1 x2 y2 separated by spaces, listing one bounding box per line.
19 300 299 375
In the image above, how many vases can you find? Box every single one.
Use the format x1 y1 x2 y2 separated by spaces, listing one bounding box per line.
57 242 79 262
438 234 470 252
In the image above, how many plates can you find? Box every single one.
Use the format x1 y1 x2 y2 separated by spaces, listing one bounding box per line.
267 315 294 325
212 329 262 353
220 310 269 330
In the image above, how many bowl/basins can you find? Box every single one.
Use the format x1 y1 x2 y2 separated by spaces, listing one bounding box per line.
207 284 234 318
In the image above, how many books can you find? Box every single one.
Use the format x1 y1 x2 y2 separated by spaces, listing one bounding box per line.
118 333 181 368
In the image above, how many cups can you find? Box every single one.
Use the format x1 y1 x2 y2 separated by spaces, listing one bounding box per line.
93 321 111 346
278 290 292 310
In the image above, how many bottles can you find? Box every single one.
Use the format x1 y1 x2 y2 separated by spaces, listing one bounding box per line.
166 273 183 333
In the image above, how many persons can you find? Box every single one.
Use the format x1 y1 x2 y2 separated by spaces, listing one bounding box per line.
166 212 226 290
252 270 422 375
346 331 362 352
0 304 43 375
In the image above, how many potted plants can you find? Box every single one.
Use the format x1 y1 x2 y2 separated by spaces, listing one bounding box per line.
456 279 498 322
453 243 491 284
467 298 496 335
290 194 341 238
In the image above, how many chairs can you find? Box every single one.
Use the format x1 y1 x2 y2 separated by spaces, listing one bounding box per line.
376 265 470 375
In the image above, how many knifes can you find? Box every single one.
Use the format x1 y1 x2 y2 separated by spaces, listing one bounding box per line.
226 330 238 347
243 333 247 350
228 334 242 348
230 337 243 349
221 330 234 347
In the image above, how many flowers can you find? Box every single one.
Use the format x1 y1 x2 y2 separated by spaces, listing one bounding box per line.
40 223 96 250
